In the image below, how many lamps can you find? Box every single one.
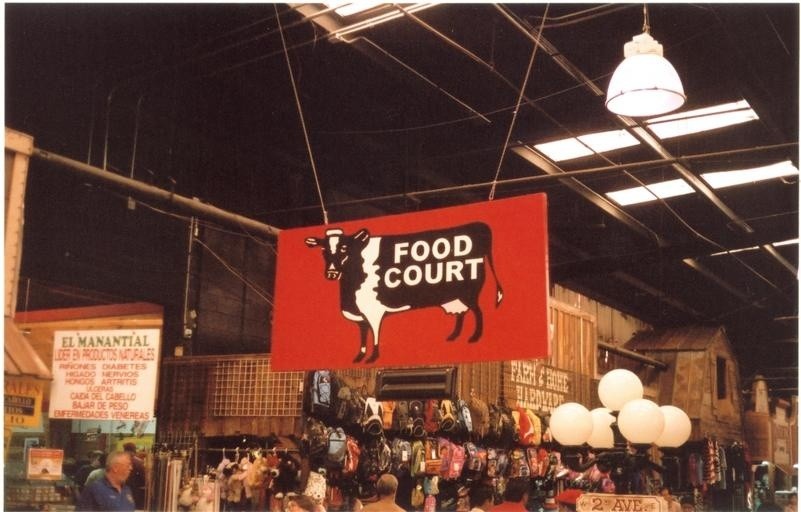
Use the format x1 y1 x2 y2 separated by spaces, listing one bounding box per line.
605 3 688 117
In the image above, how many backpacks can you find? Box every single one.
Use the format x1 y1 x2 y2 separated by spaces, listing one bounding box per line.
300 370 557 512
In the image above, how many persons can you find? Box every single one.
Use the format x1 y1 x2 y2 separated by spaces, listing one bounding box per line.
758 488 783 511
179 441 697 511
78 441 168 510
786 493 798 511
